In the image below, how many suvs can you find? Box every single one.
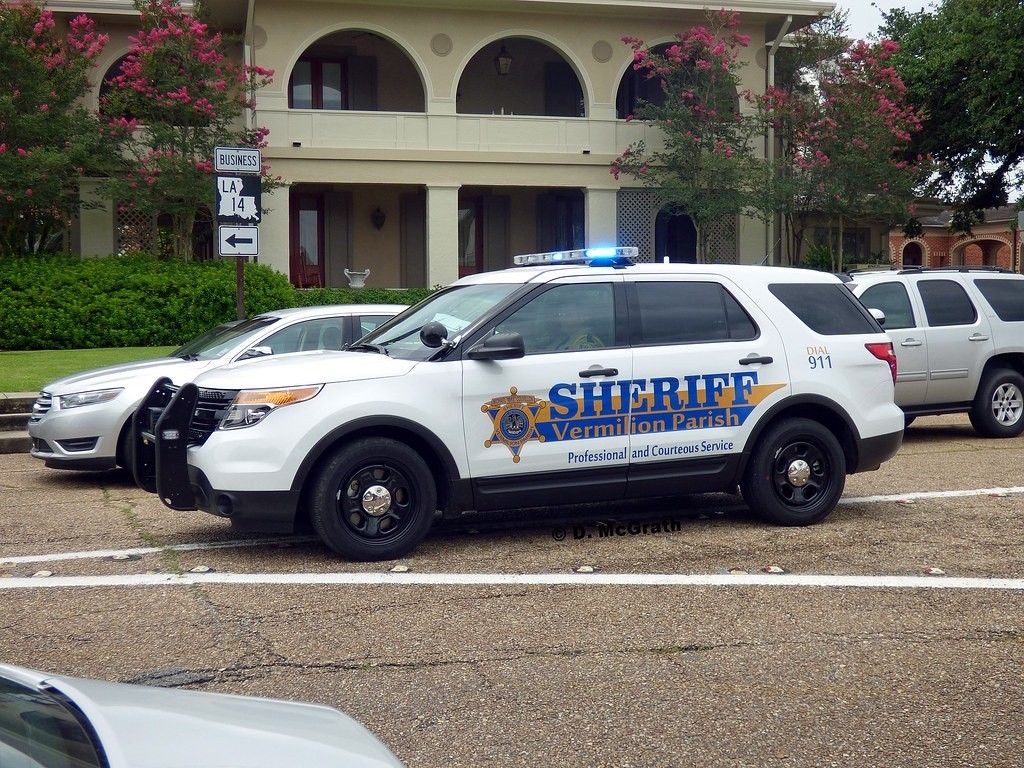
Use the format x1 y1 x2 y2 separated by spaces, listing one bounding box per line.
123 244 908 563
837 263 1024 439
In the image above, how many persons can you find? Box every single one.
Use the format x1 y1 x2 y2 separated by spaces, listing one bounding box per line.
546 298 606 350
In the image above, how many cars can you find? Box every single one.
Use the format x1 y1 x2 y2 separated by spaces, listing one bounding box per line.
18 300 505 477
0 659 408 768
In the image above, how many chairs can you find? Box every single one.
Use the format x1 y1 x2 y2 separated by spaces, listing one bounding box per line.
322 326 341 349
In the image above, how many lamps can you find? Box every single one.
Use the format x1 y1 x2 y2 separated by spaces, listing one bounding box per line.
495 38 512 75
372 206 386 230
